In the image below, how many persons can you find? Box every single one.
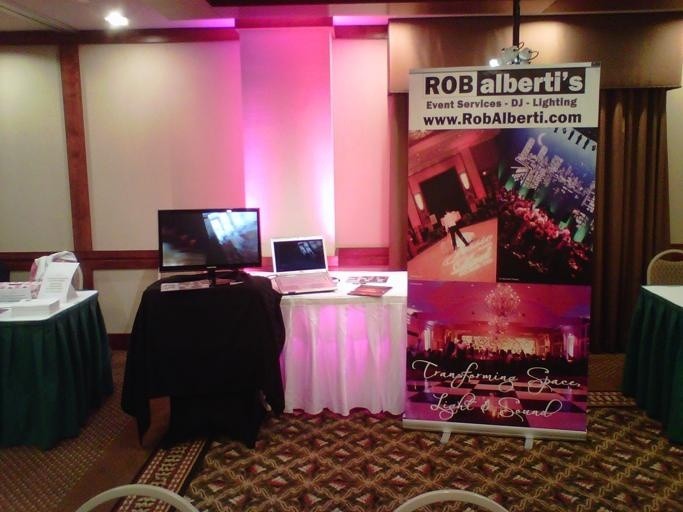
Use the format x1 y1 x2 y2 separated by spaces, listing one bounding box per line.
499 184 591 267
407 339 581 373
443 207 469 250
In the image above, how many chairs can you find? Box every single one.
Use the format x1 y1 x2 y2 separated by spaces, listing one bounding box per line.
72 480 199 511
26 256 84 290
150 273 284 450
393 486 509 512
646 246 682 284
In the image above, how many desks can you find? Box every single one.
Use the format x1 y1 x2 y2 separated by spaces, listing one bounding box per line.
138 270 409 418
619 284 683 450
0 287 114 452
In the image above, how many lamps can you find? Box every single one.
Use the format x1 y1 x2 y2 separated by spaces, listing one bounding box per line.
488 0 539 68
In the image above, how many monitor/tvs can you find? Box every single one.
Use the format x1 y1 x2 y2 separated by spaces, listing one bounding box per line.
157 208 261 291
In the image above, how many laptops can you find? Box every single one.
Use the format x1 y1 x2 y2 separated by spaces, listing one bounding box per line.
271 236 337 295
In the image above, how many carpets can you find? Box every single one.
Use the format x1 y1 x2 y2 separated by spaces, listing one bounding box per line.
109 385 683 512
